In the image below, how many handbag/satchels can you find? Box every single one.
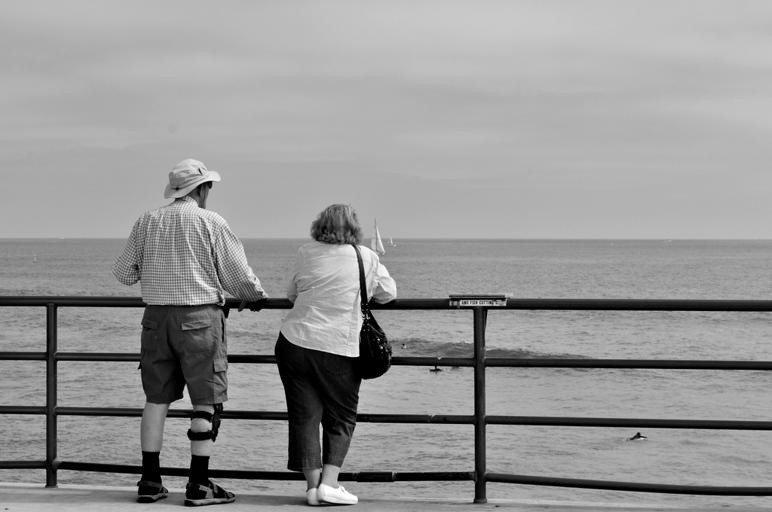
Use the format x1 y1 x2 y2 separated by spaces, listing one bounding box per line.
361 309 391 378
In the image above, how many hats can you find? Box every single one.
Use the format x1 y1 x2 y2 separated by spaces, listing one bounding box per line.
164 160 220 199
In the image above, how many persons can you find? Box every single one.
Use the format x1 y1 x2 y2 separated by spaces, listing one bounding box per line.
273 203 397 508
112 157 267 504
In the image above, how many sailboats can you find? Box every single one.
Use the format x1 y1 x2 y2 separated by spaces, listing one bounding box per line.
387 235 394 247
368 217 384 258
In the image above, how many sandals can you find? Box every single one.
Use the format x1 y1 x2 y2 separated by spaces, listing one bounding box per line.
185 480 235 506
137 480 169 503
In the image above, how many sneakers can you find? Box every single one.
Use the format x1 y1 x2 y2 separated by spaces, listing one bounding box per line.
318 483 358 504
306 488 319 506
